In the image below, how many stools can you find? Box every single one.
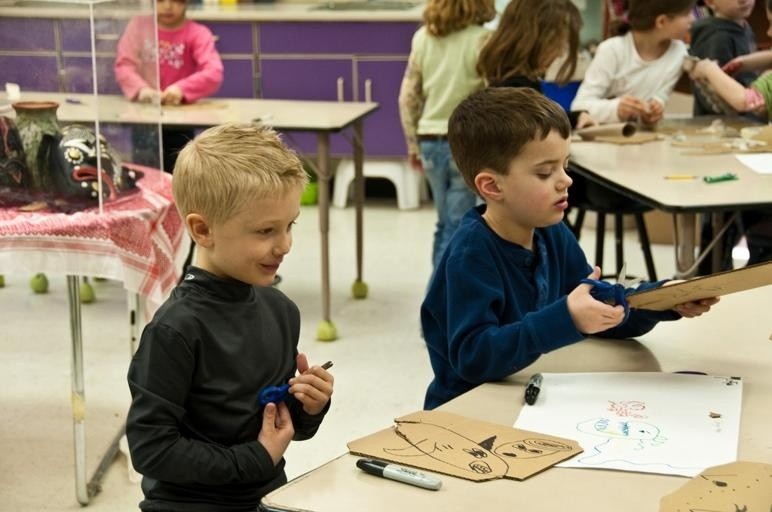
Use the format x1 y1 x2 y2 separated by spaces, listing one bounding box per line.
333 155 430 212
563 175 656 284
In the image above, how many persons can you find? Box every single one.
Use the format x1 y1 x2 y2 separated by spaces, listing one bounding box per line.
683 1 771 278
695 0 771 137
473 0 590 119
396 0 503 280
567 0 696 130
106 0 225 194
414 87 724 414
123 119 336 511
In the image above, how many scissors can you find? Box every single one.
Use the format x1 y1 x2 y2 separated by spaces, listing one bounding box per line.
258 361 334 406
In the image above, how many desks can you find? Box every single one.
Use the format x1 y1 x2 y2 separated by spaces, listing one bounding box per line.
255 277 771 512
0 160 186 505
562 110 772 282
1 88 382 344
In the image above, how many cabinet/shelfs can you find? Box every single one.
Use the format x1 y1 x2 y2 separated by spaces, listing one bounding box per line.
55 18 252 157
0 18 62 93
250 21 419 159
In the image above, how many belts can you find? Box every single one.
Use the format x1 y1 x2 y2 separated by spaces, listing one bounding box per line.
418 131 449 143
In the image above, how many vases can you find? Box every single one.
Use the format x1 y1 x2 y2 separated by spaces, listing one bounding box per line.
10 96 68 194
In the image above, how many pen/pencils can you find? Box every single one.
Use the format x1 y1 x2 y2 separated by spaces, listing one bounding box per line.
703 172 737 183
356 458 441 490
525 374 543 406
665 176 697 179
